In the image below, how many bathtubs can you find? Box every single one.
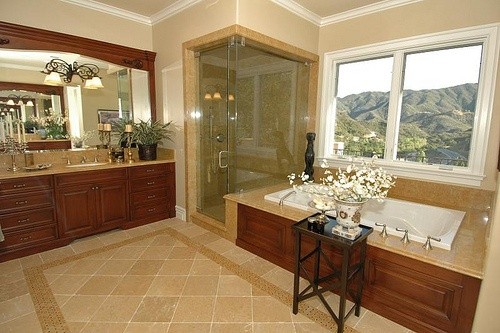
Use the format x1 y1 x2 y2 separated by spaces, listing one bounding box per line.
263 182 467 251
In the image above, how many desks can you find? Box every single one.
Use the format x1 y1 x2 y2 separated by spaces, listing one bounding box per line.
291 213 374 333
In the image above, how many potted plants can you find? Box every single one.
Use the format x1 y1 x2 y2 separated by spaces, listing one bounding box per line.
130 116 175 161
111 118 136 148
98 124 112 130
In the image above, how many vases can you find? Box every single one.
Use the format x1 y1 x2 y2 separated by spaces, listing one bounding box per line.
334 196 369 229
311 193 336 224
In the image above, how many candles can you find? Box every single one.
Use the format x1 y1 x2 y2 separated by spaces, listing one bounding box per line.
0 117 2 139
6 116 9 135
17 122 21 142
126 124 132 131
2 122 5 142
22 123 25 141
9 115 13 137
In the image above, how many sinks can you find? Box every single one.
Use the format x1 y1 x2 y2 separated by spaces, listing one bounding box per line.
66 163 108 168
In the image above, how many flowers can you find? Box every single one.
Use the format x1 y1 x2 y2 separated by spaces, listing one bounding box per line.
286 152 396 204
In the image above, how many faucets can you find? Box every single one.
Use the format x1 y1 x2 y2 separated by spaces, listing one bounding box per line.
279 191 297 205
81 155 87 163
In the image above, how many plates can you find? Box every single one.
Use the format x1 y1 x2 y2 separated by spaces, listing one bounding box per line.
25 163 52 170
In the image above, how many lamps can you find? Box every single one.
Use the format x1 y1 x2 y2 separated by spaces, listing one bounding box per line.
40 58 104 90
201 84 235 102
0 92 35 116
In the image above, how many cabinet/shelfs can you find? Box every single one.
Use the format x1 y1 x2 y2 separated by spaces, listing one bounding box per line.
235 204 480 333
0 163 176 263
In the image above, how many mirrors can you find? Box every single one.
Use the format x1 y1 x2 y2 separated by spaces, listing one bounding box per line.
0 48 152 153
0 81 72 149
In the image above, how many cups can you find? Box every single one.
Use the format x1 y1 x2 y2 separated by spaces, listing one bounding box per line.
24 153 33 166
